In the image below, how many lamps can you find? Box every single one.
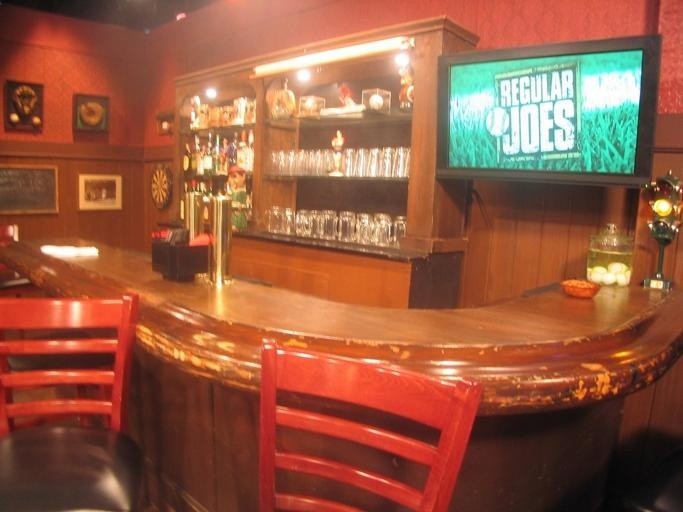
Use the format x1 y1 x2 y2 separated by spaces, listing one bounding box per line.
643 173 683 292
252 37 411 75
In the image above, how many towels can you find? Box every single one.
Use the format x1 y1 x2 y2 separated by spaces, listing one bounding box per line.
40 244 98 258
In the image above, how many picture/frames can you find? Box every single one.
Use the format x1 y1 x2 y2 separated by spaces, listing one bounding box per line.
72 92 108 138
4 81 43 132
79 174 122 211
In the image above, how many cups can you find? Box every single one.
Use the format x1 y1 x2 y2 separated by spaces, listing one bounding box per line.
268 206 406 248
269 147 410 178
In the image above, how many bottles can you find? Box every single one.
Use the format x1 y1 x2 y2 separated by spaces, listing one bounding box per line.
180 178 252 222
183 129 254 176
271 77 296 120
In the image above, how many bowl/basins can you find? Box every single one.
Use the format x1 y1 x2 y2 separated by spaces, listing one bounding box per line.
560 280 600 298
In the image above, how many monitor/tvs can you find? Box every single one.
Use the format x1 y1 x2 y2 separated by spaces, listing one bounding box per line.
436 34 661 189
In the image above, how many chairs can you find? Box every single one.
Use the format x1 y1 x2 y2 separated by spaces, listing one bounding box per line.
0 287 144 511
258 345 483 512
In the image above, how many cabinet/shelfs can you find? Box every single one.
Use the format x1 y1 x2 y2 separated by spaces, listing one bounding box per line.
230 16 481 310
173 58 256 237
